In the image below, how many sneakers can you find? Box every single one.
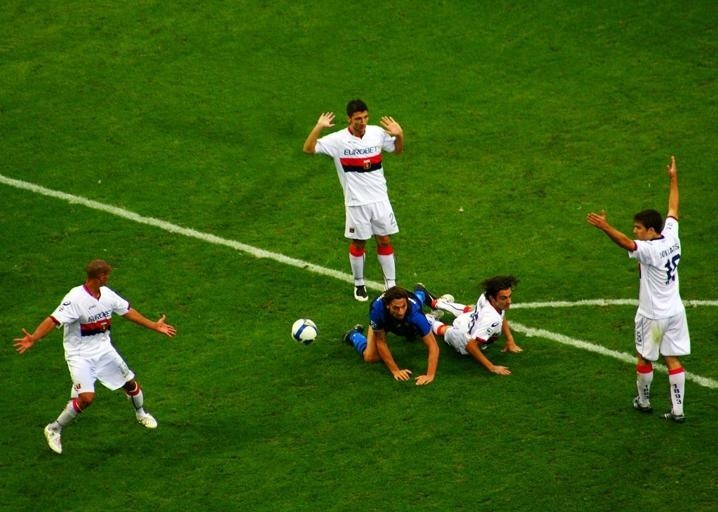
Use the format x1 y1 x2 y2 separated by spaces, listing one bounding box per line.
425 310 445 319
343 324 364 345
354 285 369 302
44 423 63 454
415 282 438 310
632 395 653 413
431 294 455 309
135 412 158 429
659 410 687 424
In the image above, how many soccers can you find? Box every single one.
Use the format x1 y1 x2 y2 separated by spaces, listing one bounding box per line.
291 318 317 345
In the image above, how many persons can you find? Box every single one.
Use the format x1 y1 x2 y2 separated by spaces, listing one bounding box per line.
587 156 691 423
344 282 439 387
424 275 523 376
12 260 176 455
304 99 403 304
